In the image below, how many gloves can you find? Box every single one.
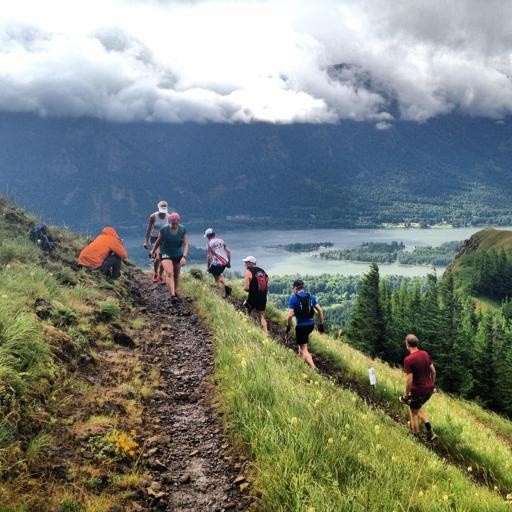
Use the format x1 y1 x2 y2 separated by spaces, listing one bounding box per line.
318 325 324 332
285 325 290 333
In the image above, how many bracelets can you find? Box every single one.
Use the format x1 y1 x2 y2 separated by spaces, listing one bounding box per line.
401 396 405 401
182 256 187 260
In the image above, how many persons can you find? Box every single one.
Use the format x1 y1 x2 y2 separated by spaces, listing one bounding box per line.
150 213 188 304
241 256 269 336
285 279 325 374
203 228 233 299
399 334 439 443
143 201 170 286
79 226 128 279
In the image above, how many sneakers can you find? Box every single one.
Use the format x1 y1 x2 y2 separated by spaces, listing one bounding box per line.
225 286 232 296
152 274 166 284
426 432 438 443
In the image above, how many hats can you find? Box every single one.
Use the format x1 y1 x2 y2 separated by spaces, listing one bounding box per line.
242 256 256 263
204 228 213 237
294 280 303 286
158 201 180 222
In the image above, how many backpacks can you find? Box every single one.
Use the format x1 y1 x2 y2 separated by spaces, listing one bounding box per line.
295 293 314 320
28 224 54 251
251 268 268 297
